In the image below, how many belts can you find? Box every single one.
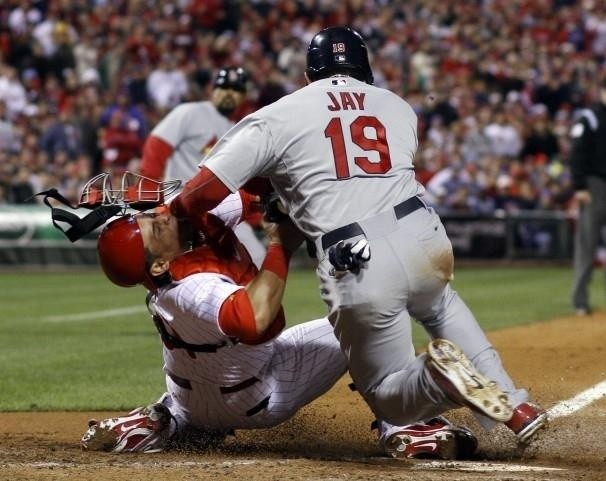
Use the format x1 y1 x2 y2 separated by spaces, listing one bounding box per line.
168 375 259 395
306 196 424 259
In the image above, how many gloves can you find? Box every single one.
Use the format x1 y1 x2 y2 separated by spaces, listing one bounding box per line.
350 238 371 262
327 240 360 276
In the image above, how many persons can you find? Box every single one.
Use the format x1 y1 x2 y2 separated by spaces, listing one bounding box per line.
137 64 270 273
168 23 548 446
0 0 606 210
78 188 478 460
567 73 606 315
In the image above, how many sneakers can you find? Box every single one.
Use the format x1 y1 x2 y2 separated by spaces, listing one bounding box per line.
385 419 478 460
504 401 549 448
425 339 514 423
80 403 171 455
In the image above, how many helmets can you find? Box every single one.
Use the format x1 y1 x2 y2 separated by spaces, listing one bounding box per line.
214 66 250 91
306 27 374 85
97 214 159 296
